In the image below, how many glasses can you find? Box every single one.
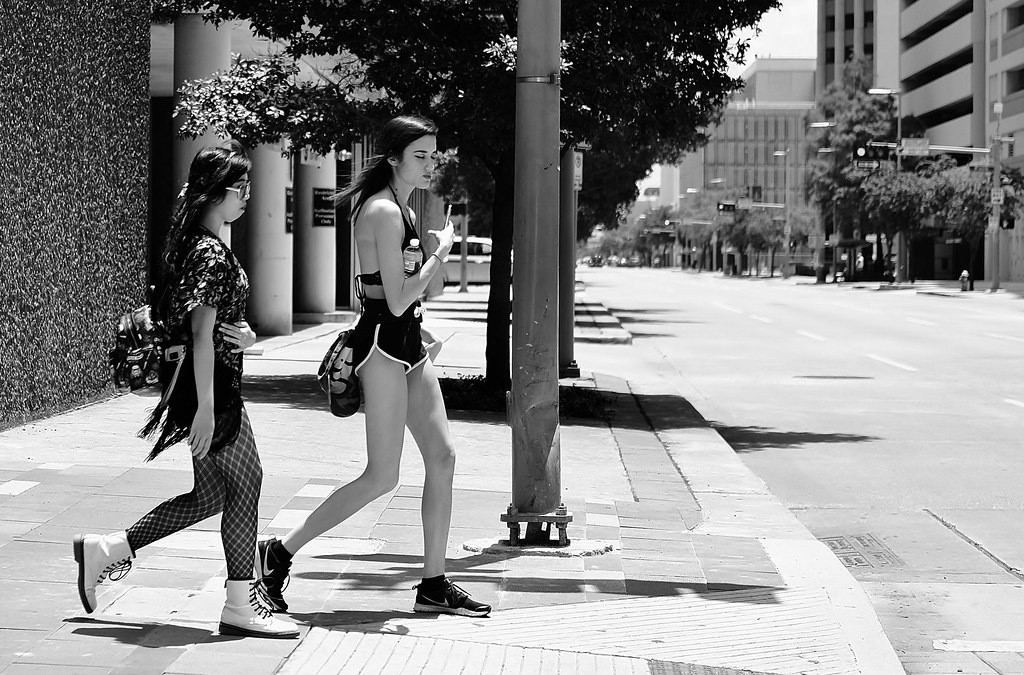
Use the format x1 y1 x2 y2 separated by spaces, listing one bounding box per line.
224 181 251 200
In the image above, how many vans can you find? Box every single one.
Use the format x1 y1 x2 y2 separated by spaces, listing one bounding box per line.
442 236 514 286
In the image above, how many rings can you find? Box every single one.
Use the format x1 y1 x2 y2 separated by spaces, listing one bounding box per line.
243 335 247 341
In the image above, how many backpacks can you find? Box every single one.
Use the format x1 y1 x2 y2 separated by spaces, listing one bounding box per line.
108 236 235 396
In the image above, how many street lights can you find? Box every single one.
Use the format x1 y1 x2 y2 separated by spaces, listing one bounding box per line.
686 188 716 271
774 149 791 280
868 88 907 284
711 178 727 270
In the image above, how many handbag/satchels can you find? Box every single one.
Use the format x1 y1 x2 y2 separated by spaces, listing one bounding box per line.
318 328 360 418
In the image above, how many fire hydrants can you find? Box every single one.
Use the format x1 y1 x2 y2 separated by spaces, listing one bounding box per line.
959 270 969 291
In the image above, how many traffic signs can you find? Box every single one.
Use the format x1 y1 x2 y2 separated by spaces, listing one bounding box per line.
855 161 881 173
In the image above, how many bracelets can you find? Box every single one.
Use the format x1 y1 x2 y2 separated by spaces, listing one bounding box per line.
427 252 443 265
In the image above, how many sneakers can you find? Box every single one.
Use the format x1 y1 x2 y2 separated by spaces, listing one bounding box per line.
412 577 492 618
254 533 292 612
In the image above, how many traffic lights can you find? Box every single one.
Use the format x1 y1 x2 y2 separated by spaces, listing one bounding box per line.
716 200 736 212
664 221 678 226
1000 213 1015 230
853 141 892 160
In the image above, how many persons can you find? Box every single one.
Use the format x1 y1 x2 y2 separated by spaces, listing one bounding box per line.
250 114 495 619
72 146 302 641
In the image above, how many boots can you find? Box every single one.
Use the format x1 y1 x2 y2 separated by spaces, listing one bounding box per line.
73 528 136 614
219 577 300 638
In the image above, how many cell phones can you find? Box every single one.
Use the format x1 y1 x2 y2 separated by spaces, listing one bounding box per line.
443 204 452 229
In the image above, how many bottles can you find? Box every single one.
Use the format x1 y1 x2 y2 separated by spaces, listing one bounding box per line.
404 238 422 280
130 363 142 379
